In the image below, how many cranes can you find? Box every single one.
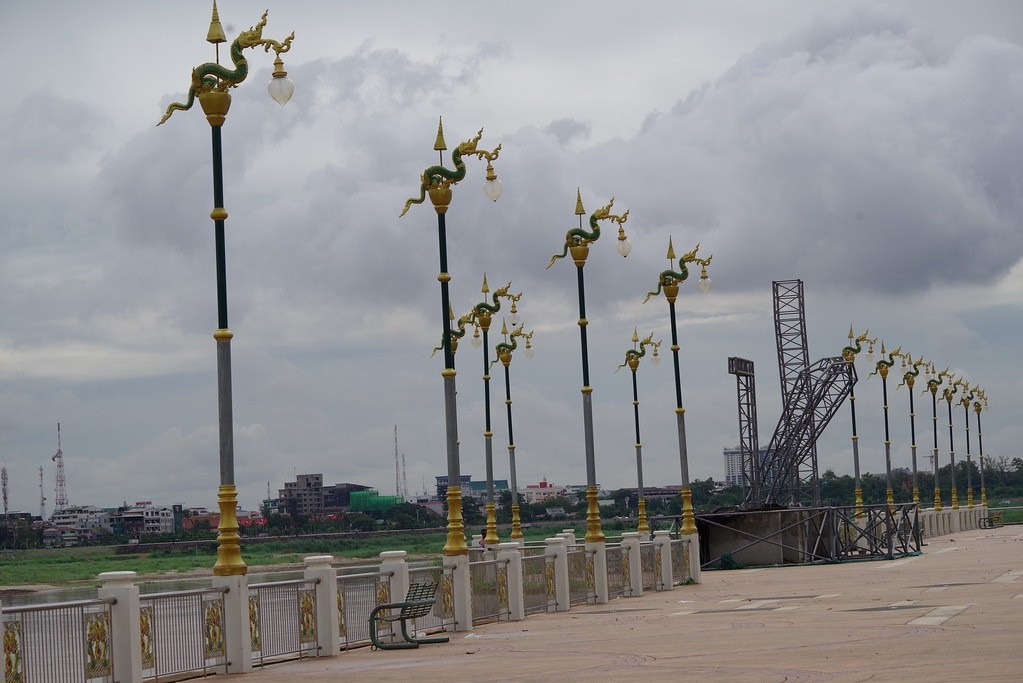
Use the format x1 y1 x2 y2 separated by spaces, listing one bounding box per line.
922 449 933 471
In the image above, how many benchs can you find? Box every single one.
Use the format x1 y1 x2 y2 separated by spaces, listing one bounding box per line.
882 516 929 548
368 580 449 650
978 510 1004 529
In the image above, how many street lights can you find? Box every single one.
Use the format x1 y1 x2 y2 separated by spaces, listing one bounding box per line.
659 241 714 536
474 282 523 545
193 8 296 576
626 331 662 533
944 376 989 509
928 370 954 512
496 323 536 540
843 330 879 518
904 361 934 514
566 198 634 545
421 127 504 556
875 345 908 515
